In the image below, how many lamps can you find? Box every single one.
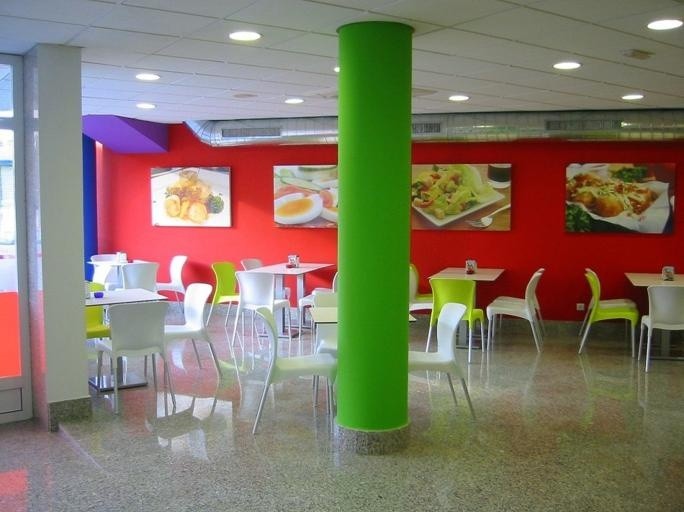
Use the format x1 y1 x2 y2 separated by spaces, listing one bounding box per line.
284 98 305 104
620 93 644 101
228 31 263 42
552 60 583 71
644 17 683 31
448 94 471 102
135 72 161 82
135 102 157 110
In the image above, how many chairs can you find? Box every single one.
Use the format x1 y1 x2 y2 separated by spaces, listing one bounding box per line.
121 262 160 291
312 292 339 408
94 302 176 415
637 285 684 373
241 259 290 300
231 270 292 348
578 272 638 359
251 307 336 436
493 268 547 343
486 272 542 352
90 253 117 261
425 278 485 363
156 255 188 313
206 262 239 327
409 263 433 311
579 267 636 336
141 283 223 385
298 272 338 341
408 302 477 421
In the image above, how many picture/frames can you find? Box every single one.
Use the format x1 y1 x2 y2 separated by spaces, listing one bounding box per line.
271 163 338 229
147 166 232 229
411 162 512 231
563 161 676 236
